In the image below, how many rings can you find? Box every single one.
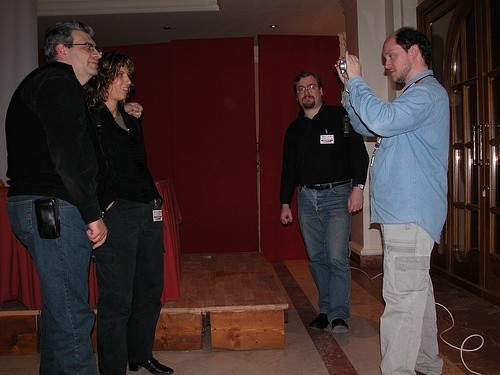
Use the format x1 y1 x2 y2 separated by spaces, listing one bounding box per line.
282 222 284 224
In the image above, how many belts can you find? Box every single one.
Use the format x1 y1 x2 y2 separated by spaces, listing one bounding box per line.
302 180 352 191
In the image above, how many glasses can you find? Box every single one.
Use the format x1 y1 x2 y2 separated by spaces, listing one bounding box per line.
297 85 318 93
65 43 103 55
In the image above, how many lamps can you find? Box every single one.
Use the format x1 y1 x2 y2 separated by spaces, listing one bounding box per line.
37 0 220 17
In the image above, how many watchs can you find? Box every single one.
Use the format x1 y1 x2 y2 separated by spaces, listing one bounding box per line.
352 182 364 190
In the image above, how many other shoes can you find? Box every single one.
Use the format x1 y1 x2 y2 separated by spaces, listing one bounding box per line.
308 313 329 329
332 318 349 333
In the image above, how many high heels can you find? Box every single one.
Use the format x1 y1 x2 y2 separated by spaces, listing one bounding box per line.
129 359 174 375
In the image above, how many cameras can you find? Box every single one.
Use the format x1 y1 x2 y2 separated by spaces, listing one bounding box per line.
339 59 347 74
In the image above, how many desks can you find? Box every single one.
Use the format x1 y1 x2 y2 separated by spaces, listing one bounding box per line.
0 178 184 311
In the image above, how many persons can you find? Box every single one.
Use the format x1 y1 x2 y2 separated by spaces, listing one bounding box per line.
279 70 370 334
85 51 175 375
4 20 142 375
334 26 450 375
